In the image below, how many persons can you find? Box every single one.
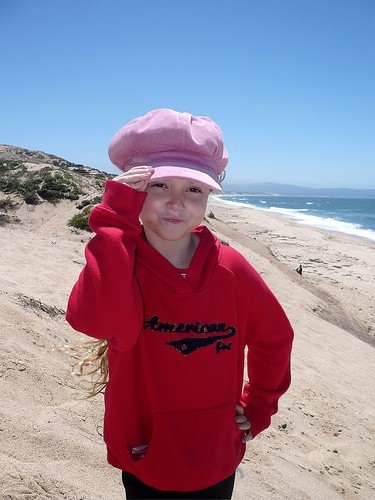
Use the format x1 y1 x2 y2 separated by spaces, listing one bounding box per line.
66 108 295 500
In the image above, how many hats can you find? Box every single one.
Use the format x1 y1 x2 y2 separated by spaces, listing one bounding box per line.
107 110 228 191
